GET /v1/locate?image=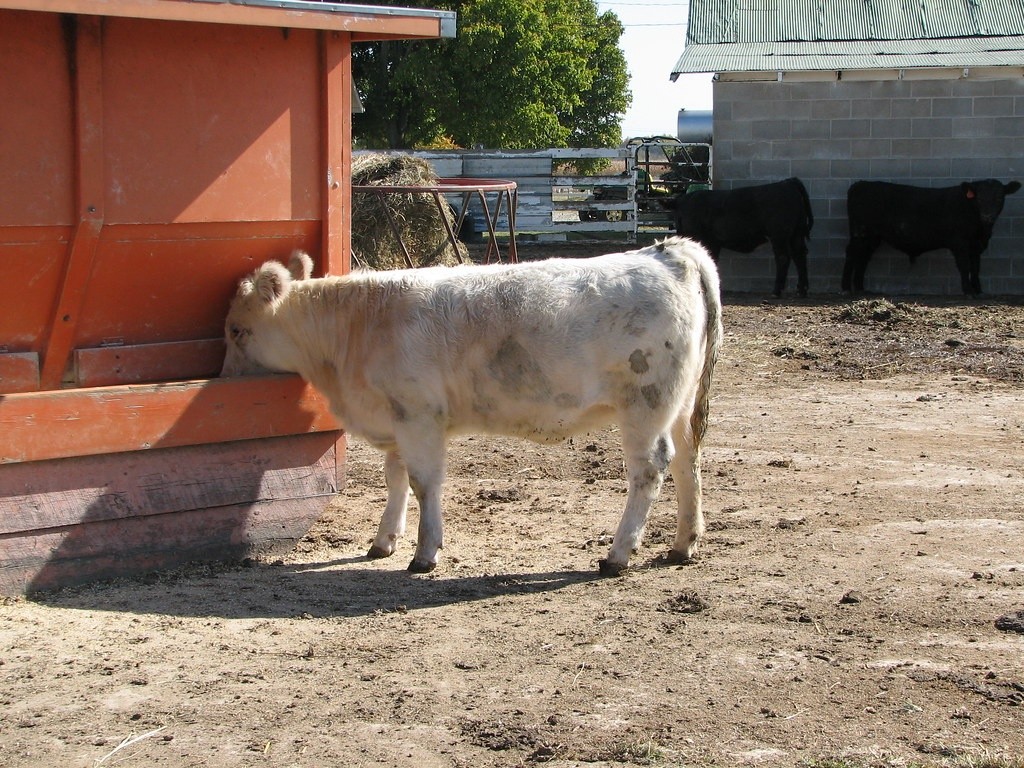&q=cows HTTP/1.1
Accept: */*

[219,236,723,576]
[657,177,813,300]
[841,179,1022,300]
